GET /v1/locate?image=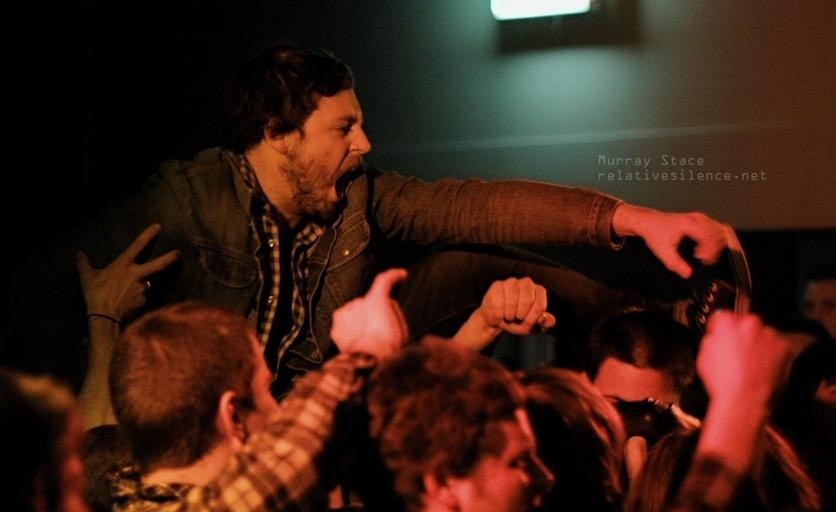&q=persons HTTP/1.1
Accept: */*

[82,35,751,403]
[0,220,836,512]
